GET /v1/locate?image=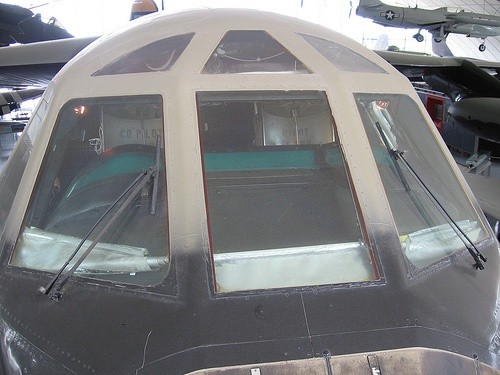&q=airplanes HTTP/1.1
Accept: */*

[1,2,497,374]
